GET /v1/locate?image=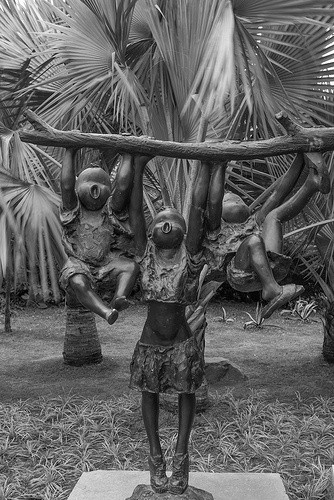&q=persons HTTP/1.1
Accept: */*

[61,129,134,325]
[129,134,218,495]
[204,139,331,320]
